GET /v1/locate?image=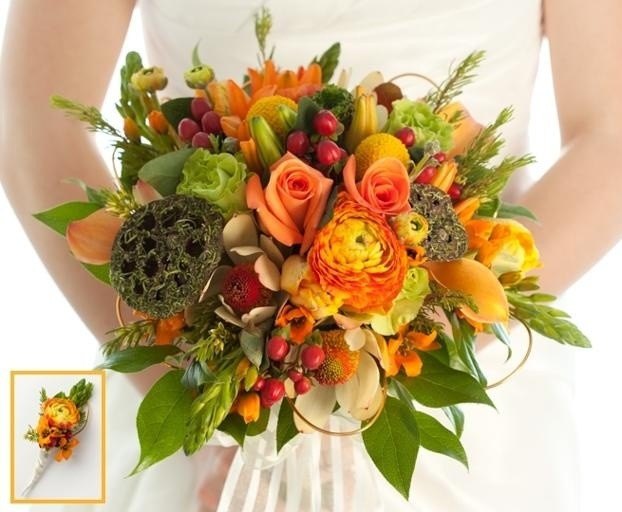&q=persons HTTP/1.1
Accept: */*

[0,2,620,511]
